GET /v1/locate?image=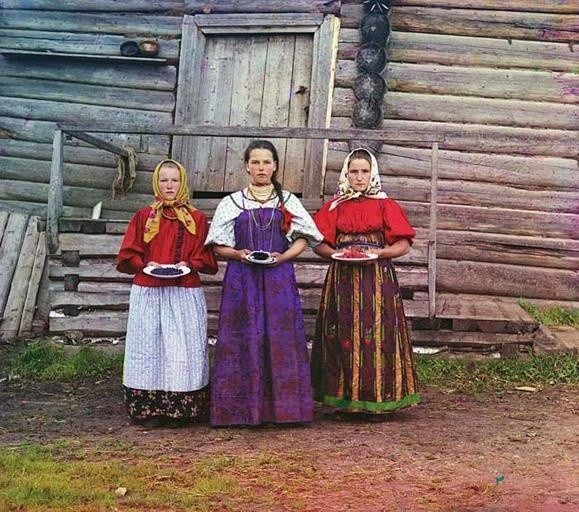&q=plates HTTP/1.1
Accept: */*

[245,254,277,265]
[330,252,378,261]
[142,264,191,279]
